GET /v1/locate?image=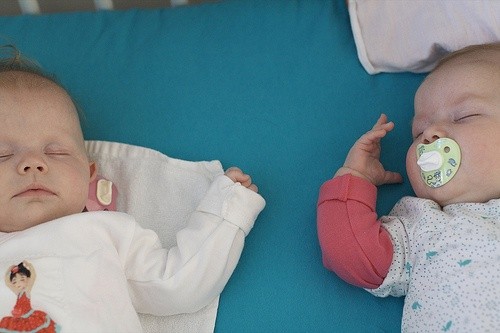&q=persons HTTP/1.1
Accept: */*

[0,43,266,333]
[316,41,500,333]
